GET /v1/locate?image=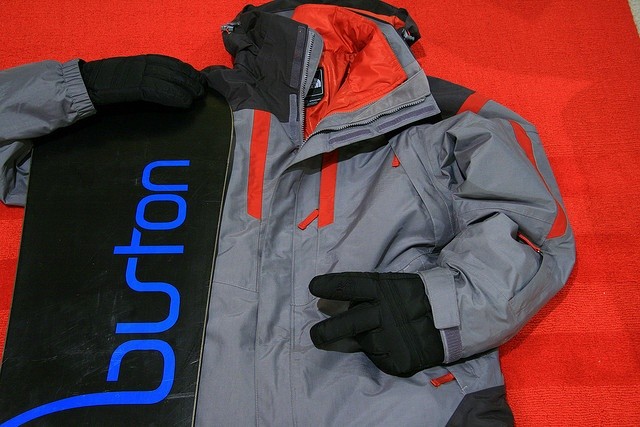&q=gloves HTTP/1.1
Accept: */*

[309,271,444,375]
[81,52,207,108]
